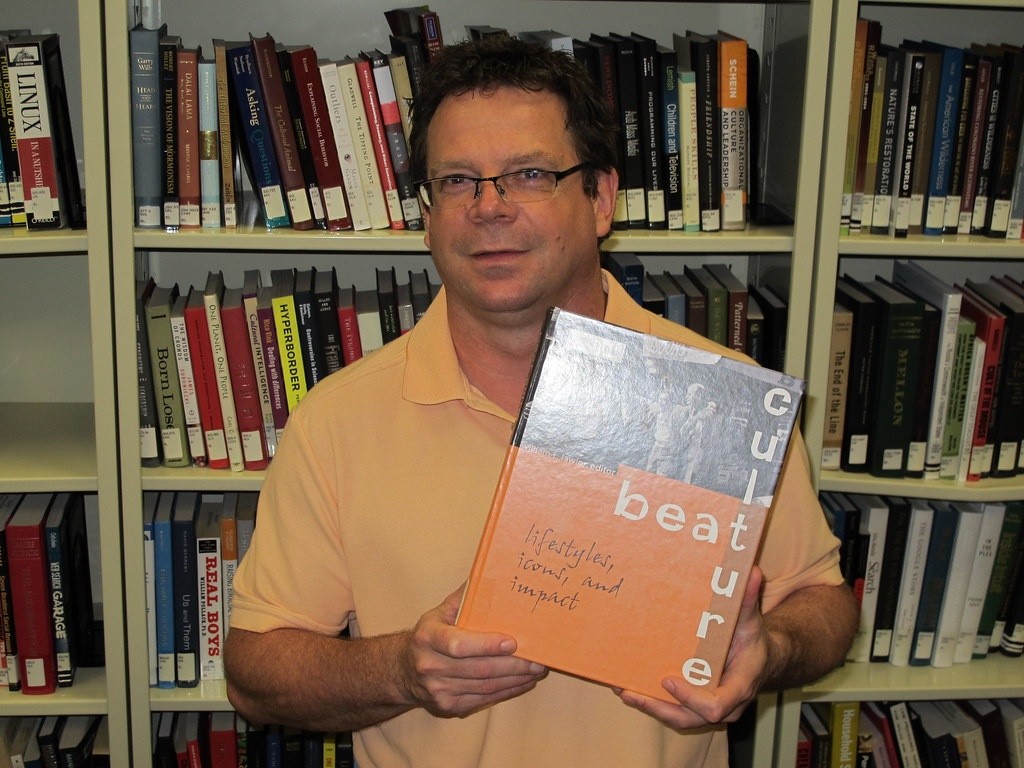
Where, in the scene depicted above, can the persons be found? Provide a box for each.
[216,28,860,768]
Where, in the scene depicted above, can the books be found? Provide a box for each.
[786,17,1024,768]
[0,19,789,768]
[449,302,808,712]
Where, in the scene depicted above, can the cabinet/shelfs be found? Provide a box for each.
[0,0,1020,768]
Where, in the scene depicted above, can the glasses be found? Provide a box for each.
[412,159,591,209]
